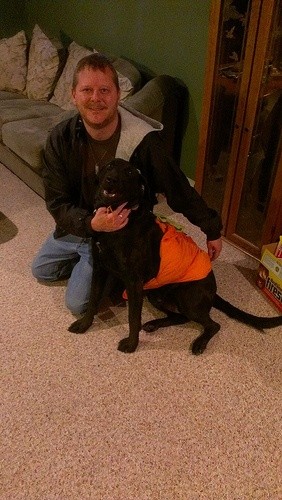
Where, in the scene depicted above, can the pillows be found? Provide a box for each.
[23,24,60,101]
[93,49,134,102]
[50,41,95,114]
[0,30,28,93]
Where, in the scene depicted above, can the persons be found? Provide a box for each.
[31,53,224,314]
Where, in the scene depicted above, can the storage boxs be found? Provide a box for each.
[256,242,282,313]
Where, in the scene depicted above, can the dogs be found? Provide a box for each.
[66,157,282,356]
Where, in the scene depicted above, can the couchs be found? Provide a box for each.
[0,27,173,200]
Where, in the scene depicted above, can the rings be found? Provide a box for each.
[119,214,123,219]
